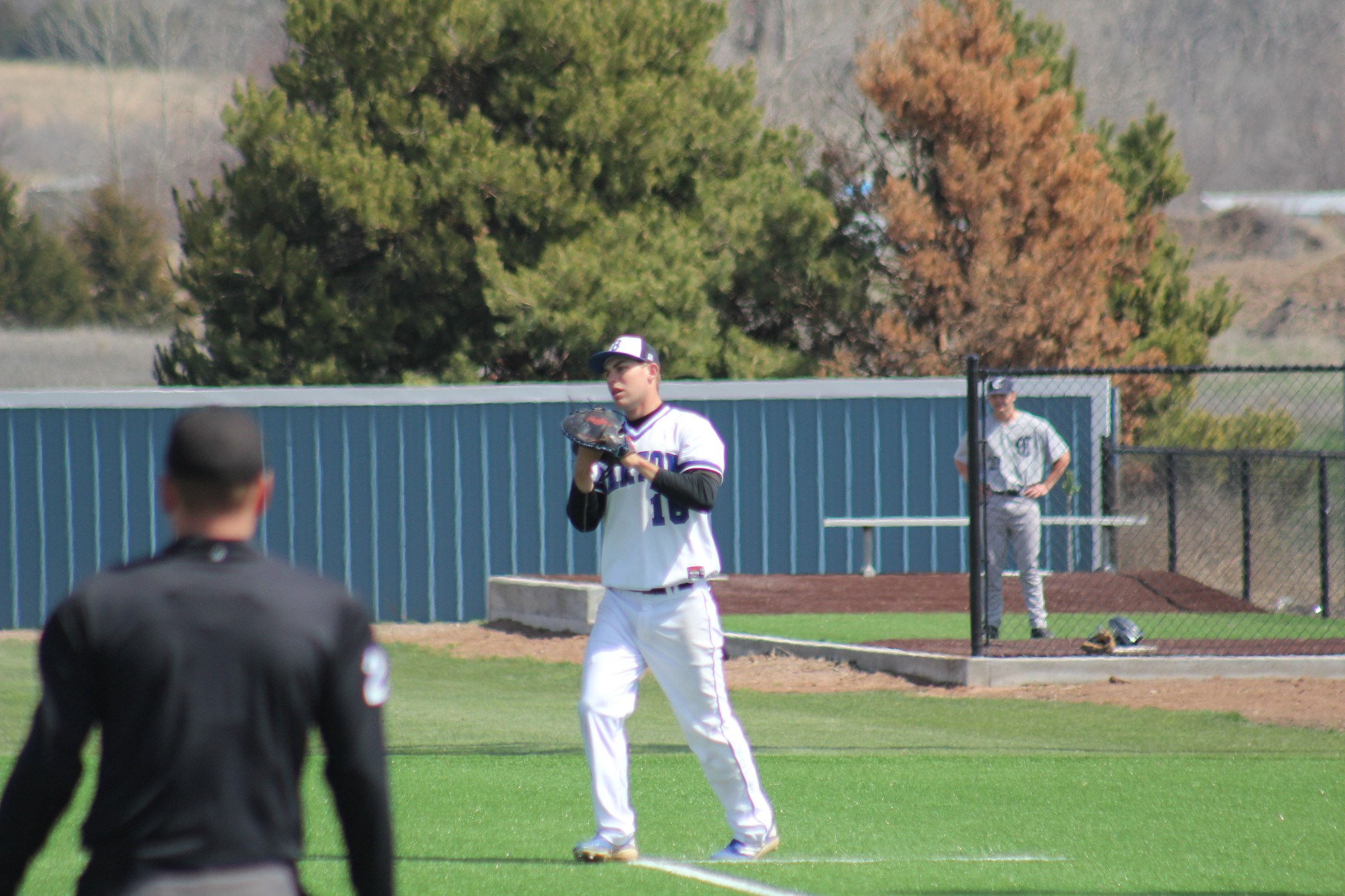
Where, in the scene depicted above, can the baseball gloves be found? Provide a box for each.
[1107,616,1145,646]
[1082,629,1114,655]
[560,396,637,459]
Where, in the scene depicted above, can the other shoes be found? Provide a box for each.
[1031,628,1054,640]
[981,625,998,640]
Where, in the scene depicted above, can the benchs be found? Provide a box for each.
[820,517,1148,577]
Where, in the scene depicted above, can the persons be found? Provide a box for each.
[565,337,779,865]
[952,376,1070,643]
[0,406,396,896]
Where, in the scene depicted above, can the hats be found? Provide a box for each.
[588,334,660,374]
[986,377,1015,396]
[165,404,263,482]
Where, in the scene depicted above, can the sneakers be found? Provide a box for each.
[572,833,638,862]
[708,827,780,862]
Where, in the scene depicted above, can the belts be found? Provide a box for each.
[993,489,1020,497]
[627,581,694,594]
